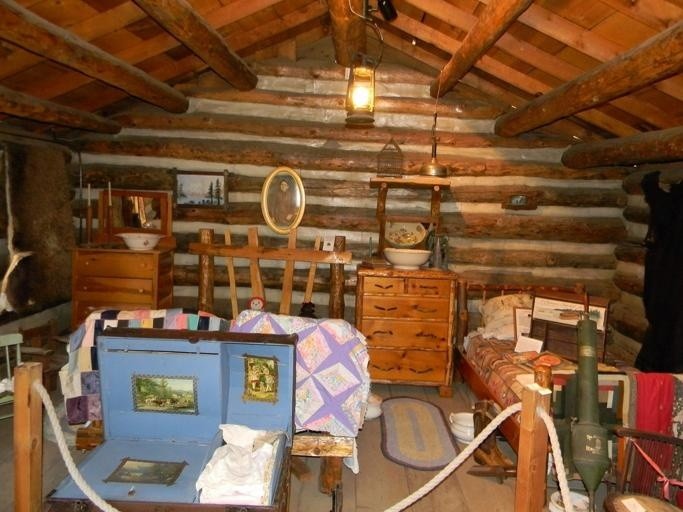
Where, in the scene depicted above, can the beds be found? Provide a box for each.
[454,277,683,512]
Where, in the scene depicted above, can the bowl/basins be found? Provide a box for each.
[383,247,433,271]
[448,412,474,445]
[365,393,383,419]
[113,232,165,251]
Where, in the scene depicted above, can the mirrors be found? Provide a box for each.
[100,189,173,240]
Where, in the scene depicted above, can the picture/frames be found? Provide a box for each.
[260,166,306,235]
[172,167,229,210]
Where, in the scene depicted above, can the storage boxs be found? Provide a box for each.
[42,327,298,511]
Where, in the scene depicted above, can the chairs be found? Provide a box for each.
[603,425,683,512]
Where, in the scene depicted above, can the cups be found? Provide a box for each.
[510,195,527,205]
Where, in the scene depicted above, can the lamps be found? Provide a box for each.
[343,11,385,130]
[419,73,447,179]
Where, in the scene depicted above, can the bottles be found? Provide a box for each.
[433,236,442,267]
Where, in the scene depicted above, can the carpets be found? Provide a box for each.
[380,393,462,473]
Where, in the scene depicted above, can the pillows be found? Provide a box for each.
[478,290,539,341]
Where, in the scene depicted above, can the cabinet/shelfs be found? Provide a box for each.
[354,177,462,398]
[70,250,174,335]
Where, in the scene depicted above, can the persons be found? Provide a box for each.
[269,178,297,226]
[248,363,260,391]
[261,362,275,392]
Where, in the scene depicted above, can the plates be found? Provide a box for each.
[384,221,426,247]
[550,490,596,512]
